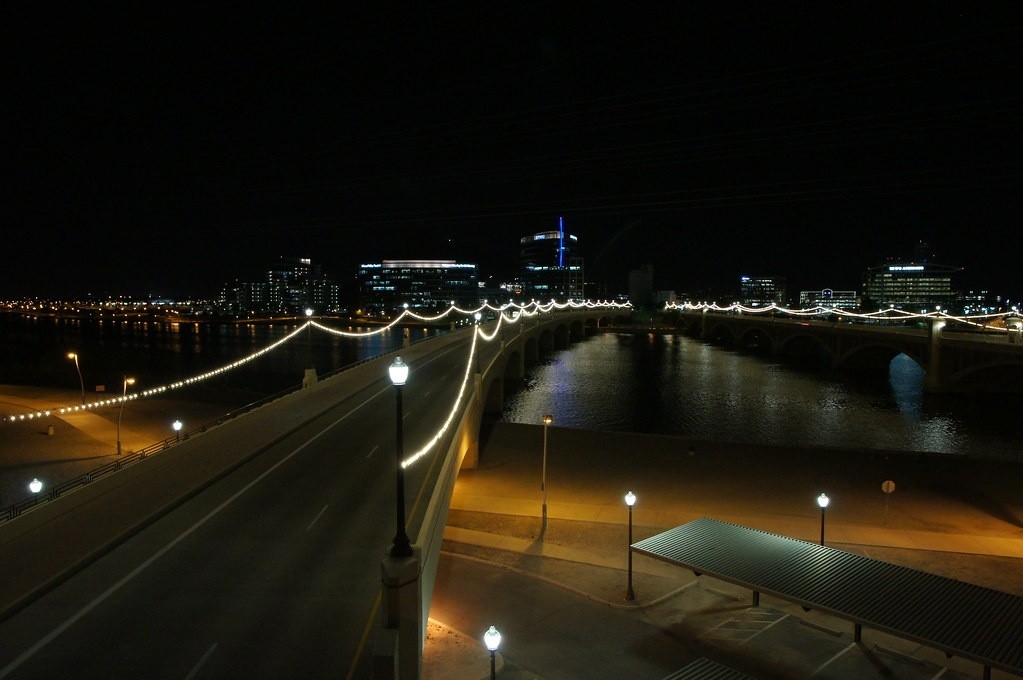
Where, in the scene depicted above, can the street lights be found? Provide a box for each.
[816,491,831,545]
[624,491,637,602]
[299,307,320,388]
[385,352,415,558]
[29,477,43,503]
[115,375,135,454]
[173,420,184,442]
[482,624,502,680]
[539,412,553,496]
[66,351,86,404]
